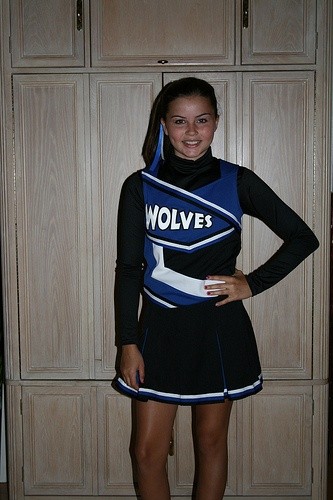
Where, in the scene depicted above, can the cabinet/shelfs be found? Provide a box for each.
[1,2,331,497]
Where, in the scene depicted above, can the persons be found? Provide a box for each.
[111,77,321,500]
